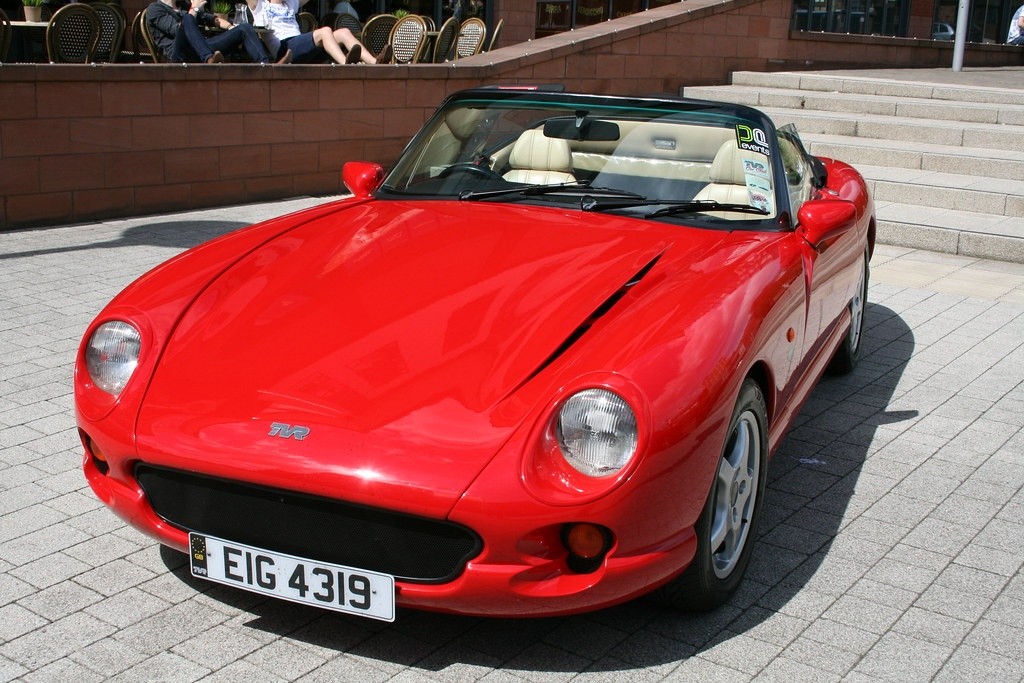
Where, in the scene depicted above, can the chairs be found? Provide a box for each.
[419,15,436,63]
[334,13,362,34]
[360,13,400,58]
[388,14,428,64]
[692,139,773,221]
[502,128,579,186]
[453,18,487,60]
[0,2,159,64]
[320,12,341,30]
[299,12,318,32]
[488,19,504,52]
[433,16,461,64]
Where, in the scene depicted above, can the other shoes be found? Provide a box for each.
[376,45,392,64]
[207,50,224,63]
[276,48,292,64]
[345,44,361,64]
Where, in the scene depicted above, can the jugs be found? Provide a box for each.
[233,4,248,26]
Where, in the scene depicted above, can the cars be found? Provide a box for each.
[931,22,956,41]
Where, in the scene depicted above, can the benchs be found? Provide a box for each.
[570,119,812,209]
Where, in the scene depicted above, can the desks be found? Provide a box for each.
[356,31,464,63]
[201,29,275,63]
[2,21,56,63]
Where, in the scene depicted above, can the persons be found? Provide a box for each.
[146,0,293,64]
[1007,5,1024,45]
[246,0,393,64]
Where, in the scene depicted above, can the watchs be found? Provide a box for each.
[190,7,200,12]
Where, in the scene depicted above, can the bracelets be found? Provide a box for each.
[227,25,232,29]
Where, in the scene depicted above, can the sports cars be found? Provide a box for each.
[74,83,878,624]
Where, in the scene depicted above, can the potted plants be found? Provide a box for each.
[212,1,234,29]
[22,0,45,22]
